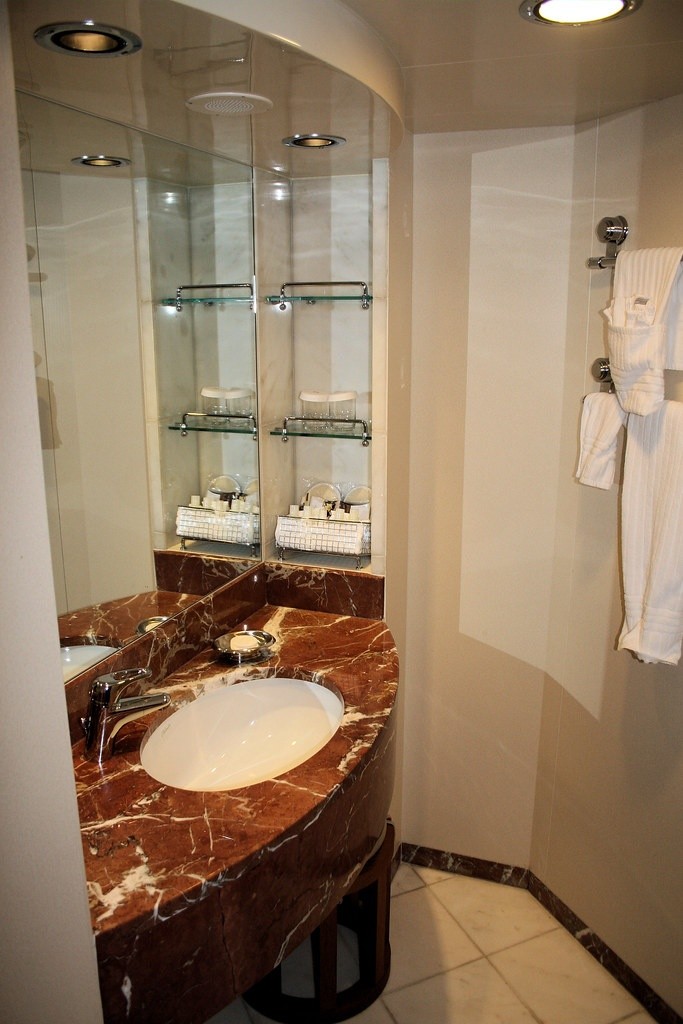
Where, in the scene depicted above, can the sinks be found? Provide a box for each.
[59,634,122,688]
[135,655,349,795]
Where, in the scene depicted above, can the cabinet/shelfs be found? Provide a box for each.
[265,281,372,447]
[158,282,257,441]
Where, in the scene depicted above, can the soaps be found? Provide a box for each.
[229,634,261,654]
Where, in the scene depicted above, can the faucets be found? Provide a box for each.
[83,666,171,762]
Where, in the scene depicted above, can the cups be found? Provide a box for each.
[302,399,329,432]
[227,395,252,428]
[203,398,226,425]
[329,398,356,432]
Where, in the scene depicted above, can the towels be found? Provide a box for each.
[575,388,627,492]
[603,244,683,668]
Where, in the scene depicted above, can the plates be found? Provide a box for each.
[343,486,372,504]
[209,476,241,500]
[242,479,258,496]
[301,483,341,518]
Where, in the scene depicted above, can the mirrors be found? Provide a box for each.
[15,86,263,679]
